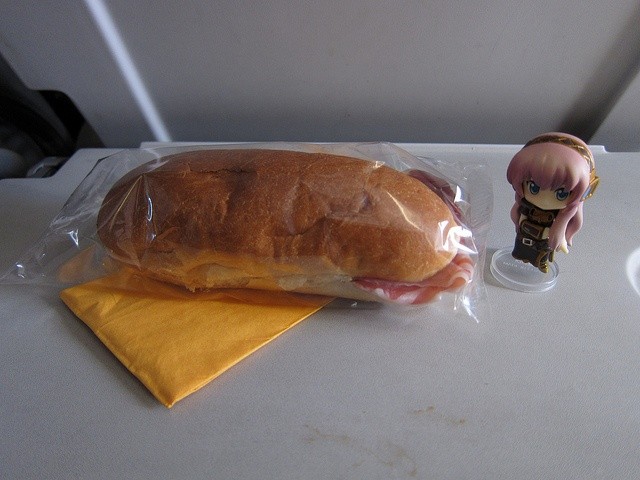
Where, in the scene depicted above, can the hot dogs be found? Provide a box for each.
[96,149,477,310]
